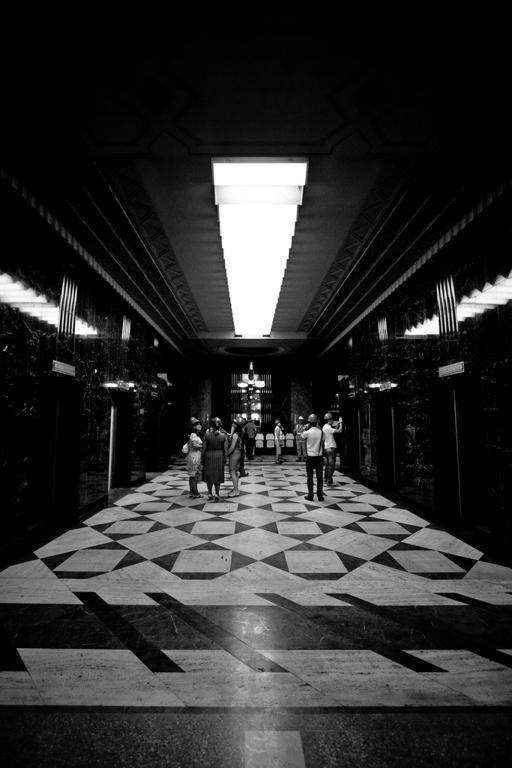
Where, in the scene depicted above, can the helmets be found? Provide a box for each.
[275,420,280,424]
[299,413,332,423]
[190,413,246,427]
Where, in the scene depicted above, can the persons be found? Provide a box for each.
[274,419,288,465]
[292,412,344,503]
[183,411,257,501]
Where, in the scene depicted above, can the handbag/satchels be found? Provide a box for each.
[181,443,190,453]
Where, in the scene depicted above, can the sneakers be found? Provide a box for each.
[318,496,324,501]
[305,495,313,501]
[190,473,248,502]
